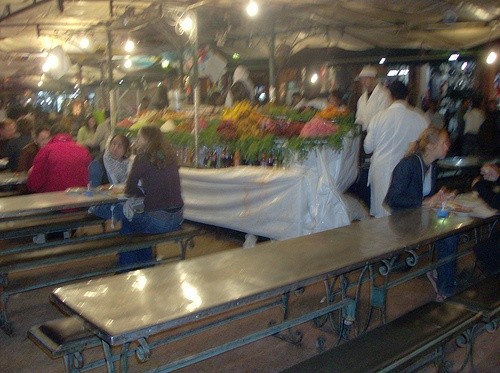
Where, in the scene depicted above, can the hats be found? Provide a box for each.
[358,63,378,77]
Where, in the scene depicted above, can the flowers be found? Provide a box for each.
[113,99,361,168]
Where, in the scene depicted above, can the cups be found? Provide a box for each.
[32,233,46,245]
[63,231,71,240]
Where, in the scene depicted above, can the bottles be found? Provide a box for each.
[176,145,285,168]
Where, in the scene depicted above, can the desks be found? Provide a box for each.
[50,184,500,373]
[0,171,128,253]
[103,124,361,241]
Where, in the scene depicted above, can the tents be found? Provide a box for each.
[0,0,500,86]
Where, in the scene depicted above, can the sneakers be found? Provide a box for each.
[33,236,46,244]
[426,269,447,298]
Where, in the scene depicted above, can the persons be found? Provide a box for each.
[87,129,134,223]
[0,61,500,303]
[23,123,94,244]
[118,127,185,273]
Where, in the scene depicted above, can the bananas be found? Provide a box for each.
[219,99,259,122]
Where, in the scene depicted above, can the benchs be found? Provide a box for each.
[27,282,499,373]
[1,223,200,336]
